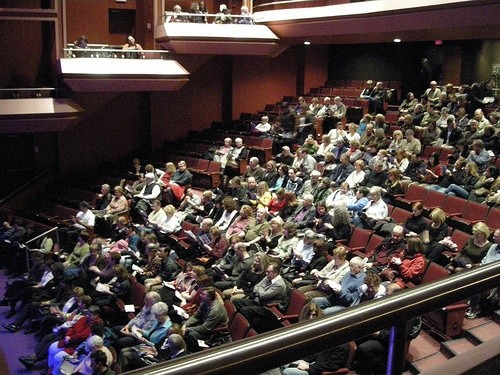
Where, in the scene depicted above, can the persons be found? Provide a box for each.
[214,4,233,24]
[234,6,254,24]
[122,36,146,59]
[0,0,500,375]
[72,36,93,58]
[190,1,209,23]
[168,5,187,23]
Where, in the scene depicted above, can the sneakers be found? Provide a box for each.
[0,298,9,306]
[23,328,35,335]
[1,323,16,333]
[19,355,37,371]
[7,274,16,279]
[5,308,16,319]
[464,307,480,320]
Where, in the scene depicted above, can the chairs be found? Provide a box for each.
[0,78,500,375]
